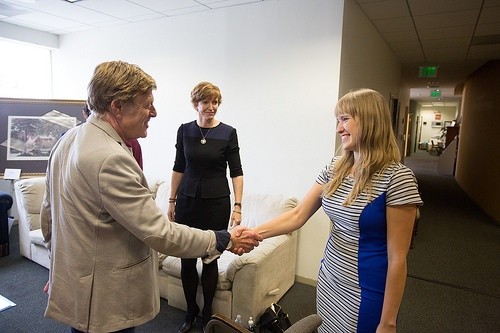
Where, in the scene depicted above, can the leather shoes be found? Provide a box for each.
[177,314,196,333]
[197,315,212,333]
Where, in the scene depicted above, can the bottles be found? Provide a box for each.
[247,316,254,333]
[235,314,243,326]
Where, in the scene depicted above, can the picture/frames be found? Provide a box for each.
[0,98,87,179]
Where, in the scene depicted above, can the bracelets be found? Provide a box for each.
[169,198,175,202]
[233,202,242,213]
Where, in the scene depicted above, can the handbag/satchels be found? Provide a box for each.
[248,303,291,333]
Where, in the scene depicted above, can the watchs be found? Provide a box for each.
[226,239,233,250]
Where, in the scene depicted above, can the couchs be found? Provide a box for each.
[14,176,299,328]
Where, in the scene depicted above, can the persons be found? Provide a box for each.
[233,88,423,333]
[83,104,143,170]
[40,61,263,332]
[168,81,243,333]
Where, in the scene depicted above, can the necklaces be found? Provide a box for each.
[196,118,214,145]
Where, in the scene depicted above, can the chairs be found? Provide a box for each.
[205,313,322,333]
